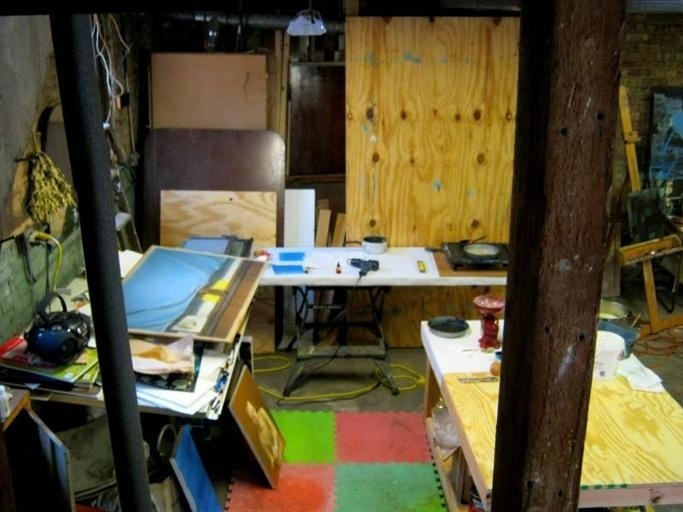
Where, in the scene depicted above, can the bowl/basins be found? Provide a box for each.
[596,319,641,359]
[362,237,389,254]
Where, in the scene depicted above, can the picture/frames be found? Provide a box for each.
[646,86,682,181]
[228,364,286,490]
[121,245,265,344]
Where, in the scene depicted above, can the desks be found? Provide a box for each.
[420,320,683,512]
[0,249,251,418]
[251,246,513,395]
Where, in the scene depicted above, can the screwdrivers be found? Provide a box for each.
[336,261,341,273]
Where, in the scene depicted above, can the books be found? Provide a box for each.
[0,336,103,402]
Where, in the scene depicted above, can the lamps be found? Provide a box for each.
[286,0,326,37]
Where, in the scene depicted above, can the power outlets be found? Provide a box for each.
[17,227,36,254]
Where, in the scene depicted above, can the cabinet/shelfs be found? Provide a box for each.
[0,389,73,512]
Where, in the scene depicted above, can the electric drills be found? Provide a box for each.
[346,258,378,276]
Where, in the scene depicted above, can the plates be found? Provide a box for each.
[428,315,471,339]
